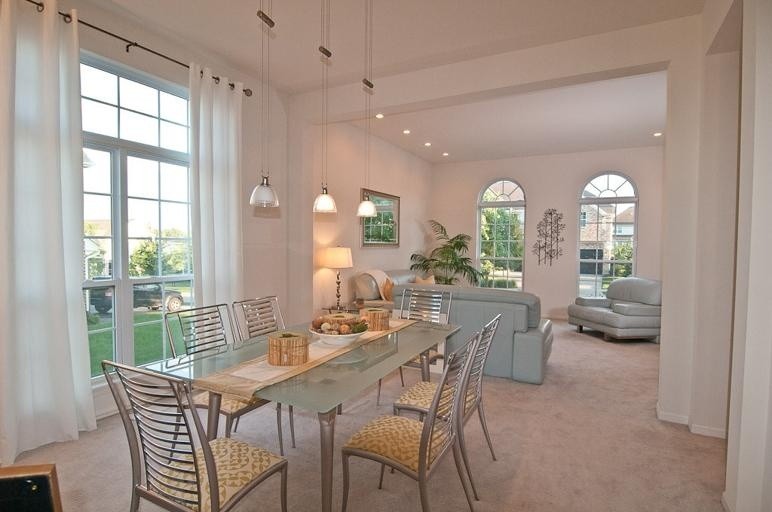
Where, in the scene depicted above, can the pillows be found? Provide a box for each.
[384,278,393,301]
[415,275,436,285]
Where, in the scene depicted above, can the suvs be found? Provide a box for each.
[89,275,183,313]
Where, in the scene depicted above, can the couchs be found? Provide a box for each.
[353,269,430,311]
[381,282,554,386]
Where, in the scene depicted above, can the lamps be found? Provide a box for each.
[356,1,378,218]
[325,244,354,310]
[249,1,281,209]
[313,1,339,215]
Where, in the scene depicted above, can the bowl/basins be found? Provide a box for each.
[308,328,367,347]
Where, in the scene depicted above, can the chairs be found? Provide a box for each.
[566,275,662,342]
